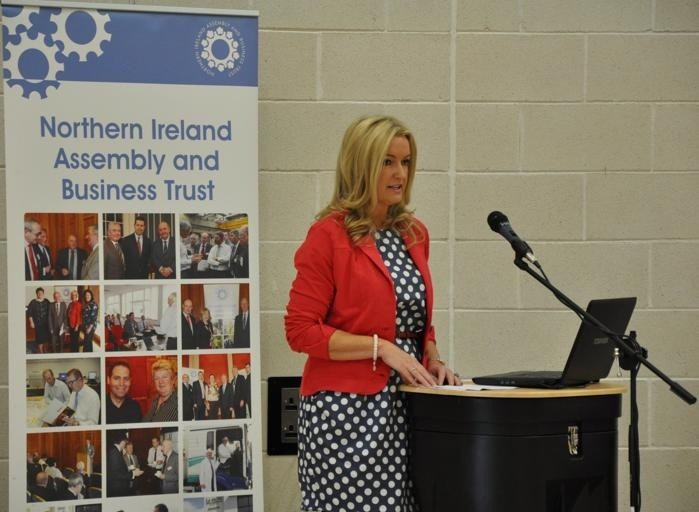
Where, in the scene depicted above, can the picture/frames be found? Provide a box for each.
[267,376,303,455]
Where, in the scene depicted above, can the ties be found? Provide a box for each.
[164,240,167,253]
[43,247,49,266]
[201,244,205,257]
[154,449,157,461]
[28,243,39,280]
[115,243,123,264]
[70,250,74,272]
[187,315,191,329]
[138,237,141,256]
[243,314,245,329]
[130,456,133,465]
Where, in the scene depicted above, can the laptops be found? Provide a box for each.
[472,295,639,389]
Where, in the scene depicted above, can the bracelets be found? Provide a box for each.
[373,334,378,371]
[429,356,447,365]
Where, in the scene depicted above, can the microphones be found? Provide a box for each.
[487,211,541,269]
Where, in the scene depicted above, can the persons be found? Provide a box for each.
[104,293,177,352]
[102,218,176,279]
[42,368,100,427]
[141,359,178,422]
[105,361,142,424]
[182,363,251,422]
[27,439,96,502]
[24,218,99,280]
[180,221,249,278]
[284,115,462,512]
[28,287,99,354]
[106,429,179,497]
[181,298,250,349]
[182,430,251,494]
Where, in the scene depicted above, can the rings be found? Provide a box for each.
[454,372,460,377]
[409,368,415,372]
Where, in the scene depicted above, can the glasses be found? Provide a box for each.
[66,380,78,386]
